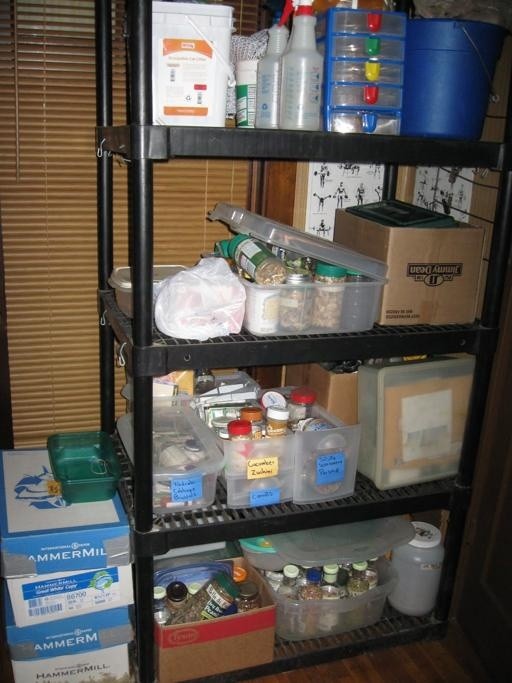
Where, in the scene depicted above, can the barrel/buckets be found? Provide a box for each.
[403,18,497,135]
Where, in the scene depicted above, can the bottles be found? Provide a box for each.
[227,234,371,338]
[275,556,380,636]
[211,385,343,504]
[386,520,446,622]
[152,572,260,644]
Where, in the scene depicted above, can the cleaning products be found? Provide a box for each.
[257,21,287,128]
[277,0,323,131]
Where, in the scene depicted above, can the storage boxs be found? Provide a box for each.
[0,435,137,682]
[153,513,416,683]
[117,352,478,514]
[108,203,485,343]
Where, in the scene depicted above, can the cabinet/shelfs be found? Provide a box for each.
[86,0,512,683]
